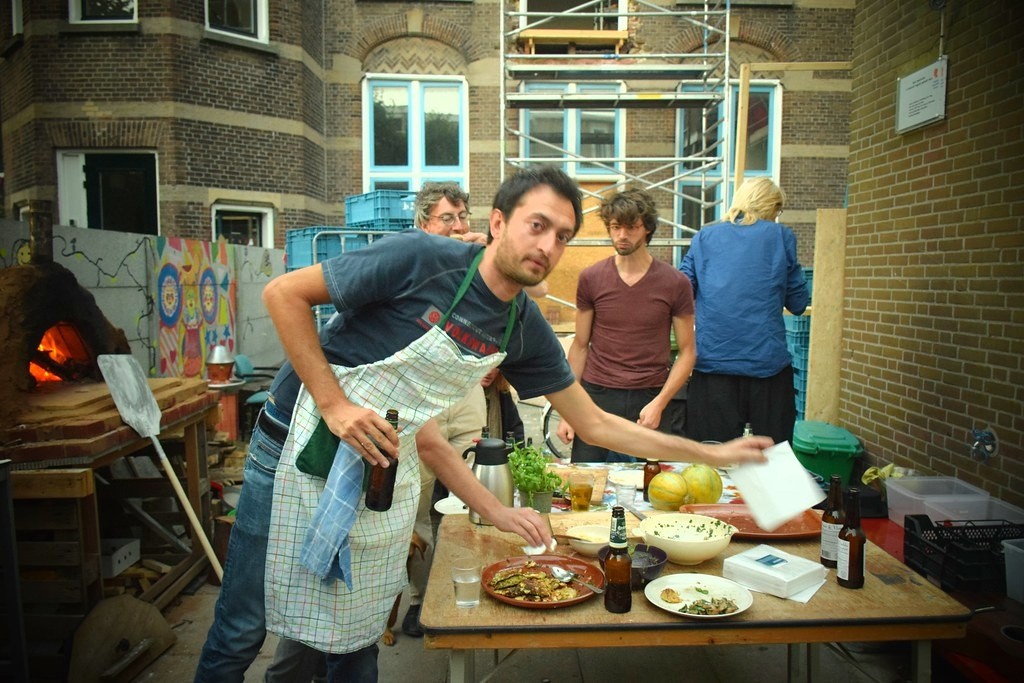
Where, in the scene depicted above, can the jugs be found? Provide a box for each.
[462,438,515,525]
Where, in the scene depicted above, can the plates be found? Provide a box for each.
[480,555,605,608]
[607,470,646,489]
[644,573,753,617]
[716,466,734,471]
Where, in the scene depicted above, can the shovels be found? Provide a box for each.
[97,354,224,586]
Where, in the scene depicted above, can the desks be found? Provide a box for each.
[0,382,221,628]
[417,455,972,683]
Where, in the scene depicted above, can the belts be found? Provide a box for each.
[258,410,289,446]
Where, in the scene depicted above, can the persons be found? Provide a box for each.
[193,169,775,683]
[677,177,809,449]
[380,182,553,646]
[556,188,697,464]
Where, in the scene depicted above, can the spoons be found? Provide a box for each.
[551,565,604,593]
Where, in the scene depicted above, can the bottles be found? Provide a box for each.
[604,507,632,613]
[643,457,661,503]
[820,473,848,567]
[837,488,866,589]
[364,409,398,512]
[742,421,754,436]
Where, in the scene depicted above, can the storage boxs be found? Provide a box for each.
[66,537,141,578]
[283,188,421,340]
[999,540,1024,602]
[881,470,989,525]
[898,511,1024,606]
[782,312,811,419]
[802,267,812,300]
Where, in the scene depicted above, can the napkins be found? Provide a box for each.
[726,440,825,533]
[725,542,827,606]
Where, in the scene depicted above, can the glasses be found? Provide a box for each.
[777,207,784,216]
[429,210,473,225]
[606,223,644,232]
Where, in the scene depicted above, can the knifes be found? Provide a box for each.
[620,501,647,521]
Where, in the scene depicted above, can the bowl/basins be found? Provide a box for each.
[640,514,740,565]
[565,525,610,558]
[597,543,668,590]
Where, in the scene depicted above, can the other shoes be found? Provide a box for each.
[401,604,424,637]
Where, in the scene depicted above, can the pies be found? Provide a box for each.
[488,567,576,604]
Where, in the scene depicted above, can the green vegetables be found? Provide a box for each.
[653,519,732,538]
[679,587,726,615]
[507,438,569,506]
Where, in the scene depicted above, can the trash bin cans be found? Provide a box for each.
[792,420,864,486]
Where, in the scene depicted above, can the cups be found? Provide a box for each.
[451,558,482,608]
[568,473,593,512]
[616,483,636,512]
[701,440,722,445]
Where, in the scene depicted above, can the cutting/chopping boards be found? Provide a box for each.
[546,468,609,505]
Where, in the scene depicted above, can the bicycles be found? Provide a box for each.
[539,401,573,459]
